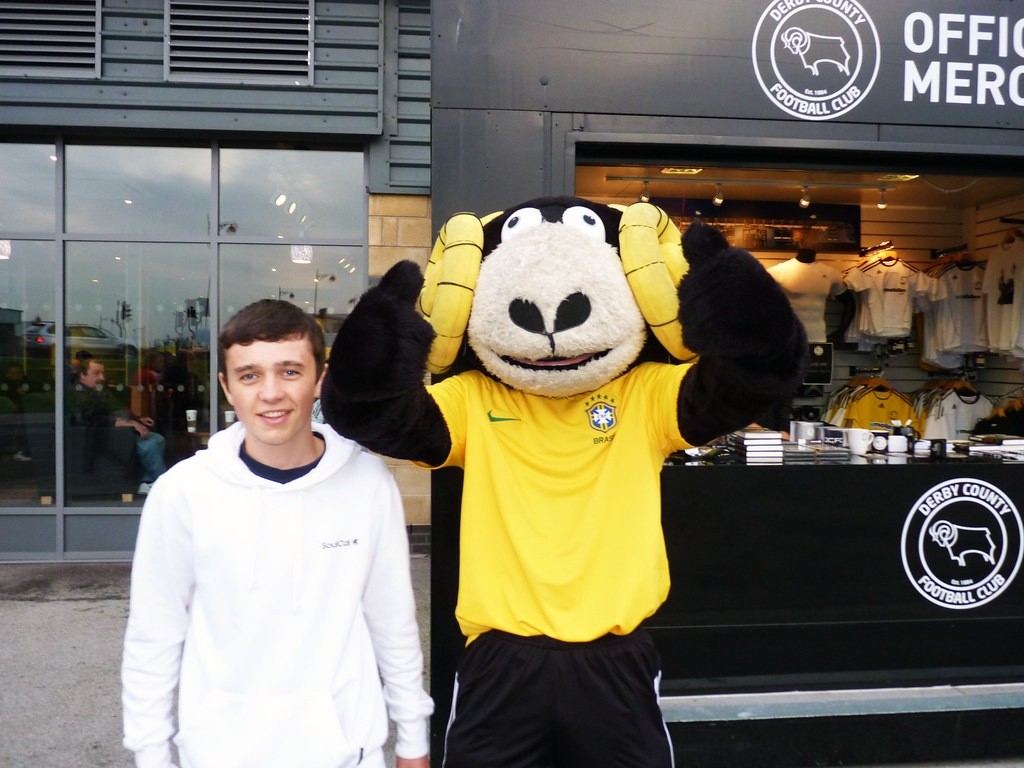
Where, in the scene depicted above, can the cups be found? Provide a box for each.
[849,429,874,455]
[186,409,197,433]
[870,430,890,453]
[224,411,236,428]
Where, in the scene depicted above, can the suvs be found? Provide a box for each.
[21,321,138,363]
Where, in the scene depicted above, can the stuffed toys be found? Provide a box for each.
[321,202,809,768]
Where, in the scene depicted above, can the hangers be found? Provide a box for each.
[878,241,897,259]
[935,372,977,395]
[846,366,893,392]
[988,389,1024,420]
[1002,219,1024,245]
[944,243,973,266]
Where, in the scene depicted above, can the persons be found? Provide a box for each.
[121,299,437,768]
[66,360,167,495]
[0,344,94,462]
[128,350,167,424]
[765,249,844,344]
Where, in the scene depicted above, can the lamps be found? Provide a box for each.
[878,188,887,209]
[712,184,724,206]
[799,186,811,209]
[641,182,649,202]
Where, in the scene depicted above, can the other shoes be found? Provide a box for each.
[138,483,151,494]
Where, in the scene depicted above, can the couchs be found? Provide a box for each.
[25,393,141,504]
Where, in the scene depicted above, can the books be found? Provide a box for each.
[726,425,849,465]
